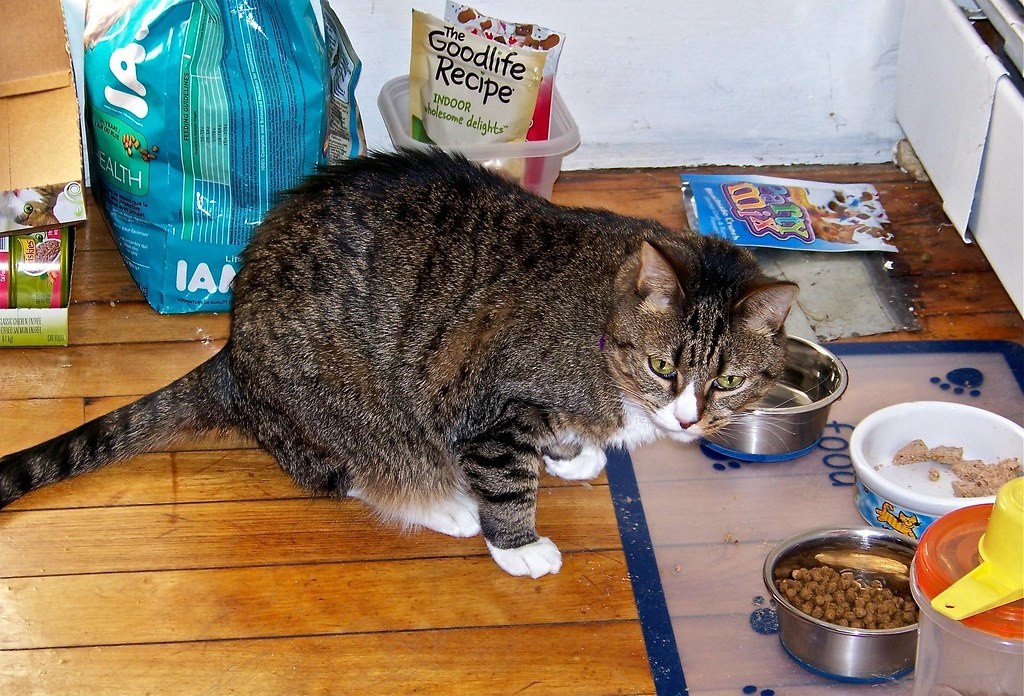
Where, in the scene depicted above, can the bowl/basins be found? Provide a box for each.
[698,336,848,462]
[850,402,1023,538]
[765,527,921,683]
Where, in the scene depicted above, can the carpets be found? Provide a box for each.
[606,336,1024,696]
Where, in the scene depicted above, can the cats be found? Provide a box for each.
[0,146,796,579]
[0,180,81,231]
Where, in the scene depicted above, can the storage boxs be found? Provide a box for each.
[380,72,580,201]
[0,0,91,348]
[887,0,1024,320]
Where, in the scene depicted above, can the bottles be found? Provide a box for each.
[909,503,1024,696]
